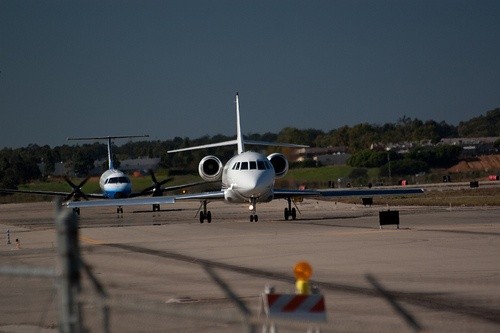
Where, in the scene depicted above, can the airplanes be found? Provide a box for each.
[63,92,427,224]
[2,132,223,215]
